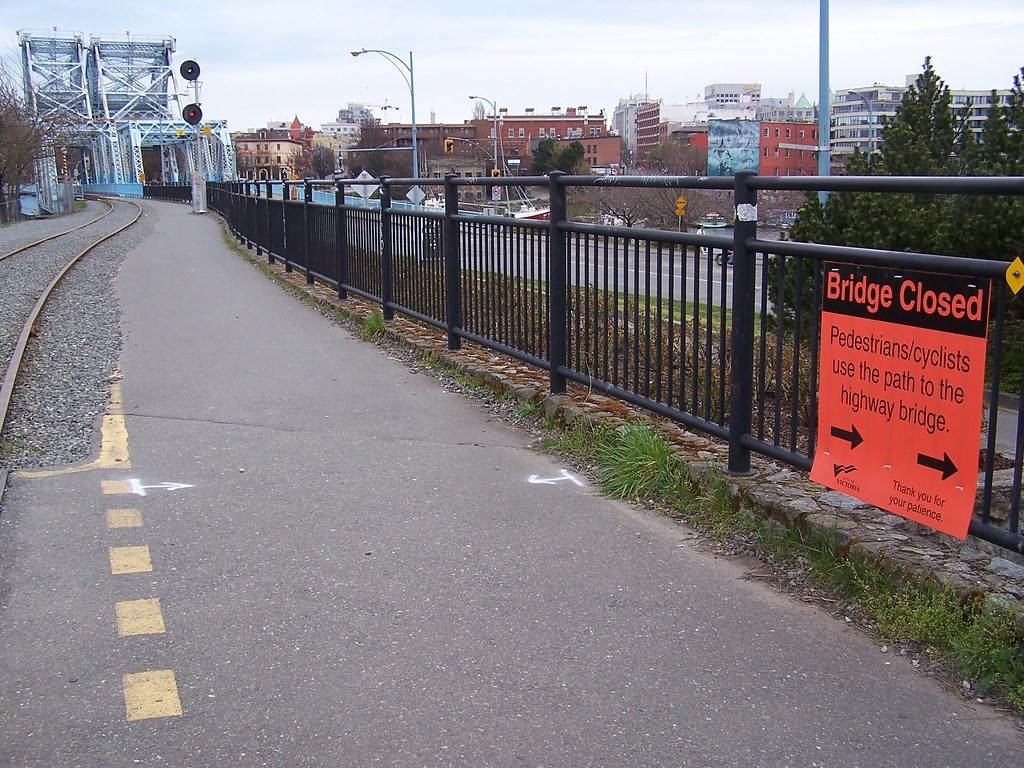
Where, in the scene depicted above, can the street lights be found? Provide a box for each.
[468,95,498,167]
[351,48,419,177]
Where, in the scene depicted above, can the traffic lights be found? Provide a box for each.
[492,170,501,178]
[181,61,201,81]
[182,104,203,125]
[445,139,454,154]
